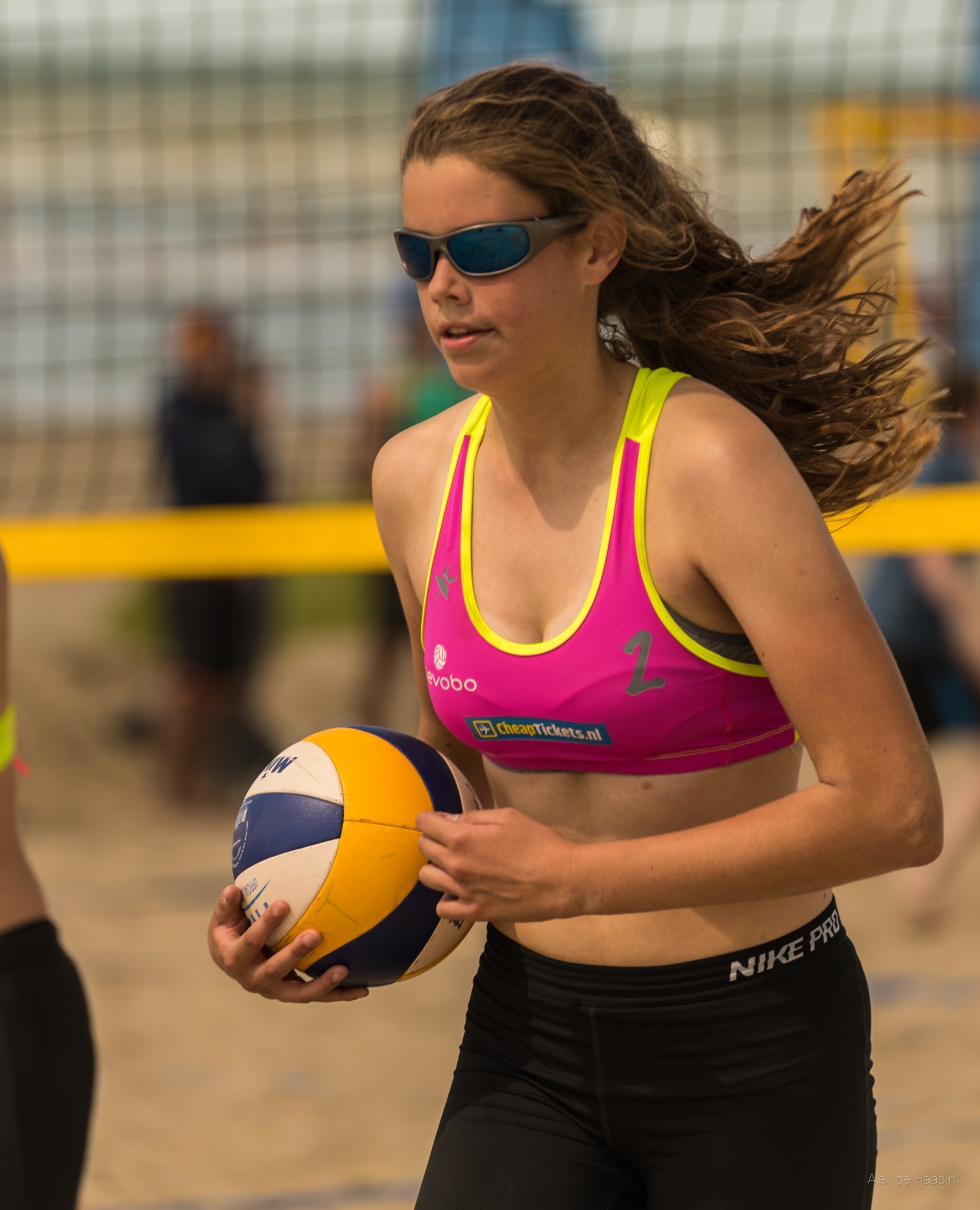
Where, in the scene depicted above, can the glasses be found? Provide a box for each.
[391,215,595,284]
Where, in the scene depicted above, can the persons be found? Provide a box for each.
[154,280,484,822]
[0,559,95,1210]
[845,355,980,924]
[206,62,946,1209]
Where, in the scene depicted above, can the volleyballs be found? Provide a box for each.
[230,726,485,985]
